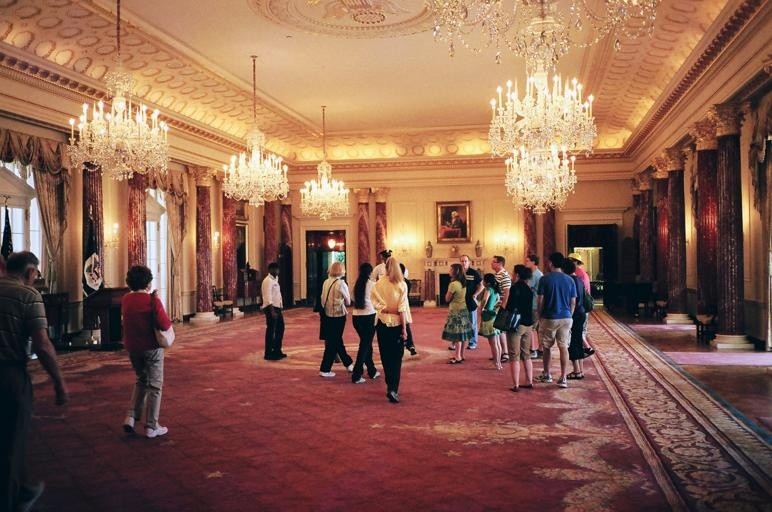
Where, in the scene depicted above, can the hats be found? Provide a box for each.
[567,253,583,264]
[380,250,392,257]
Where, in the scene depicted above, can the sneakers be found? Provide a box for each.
[347,363,380,383]
[530,349,543,358]
[582,347,594,358]
[122,416,134,432]
[145,424,168,438]
[533,374,553,383]
[20,480,45,510]
[320,370,335,378]
[556,376,567,388]
[387,391,398,402]
[265,354,287,360]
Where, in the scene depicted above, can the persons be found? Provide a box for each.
[118,264,176,442]
[370,256,411,402]
[437,250,597,395]
[349,263,386,385]
[320,262,356,377]
[259,261,289,360]
[312,270,351,363]
[2,249,67,510]
[394,262,419,361]
[368,247,392,278]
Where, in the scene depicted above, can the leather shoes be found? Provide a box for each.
[510,386,519,390]
[519,384,533,388]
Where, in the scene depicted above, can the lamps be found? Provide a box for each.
[68,0,171,181]
[219,56,289,208]
[299,105,350,220]
[428,1,660,216]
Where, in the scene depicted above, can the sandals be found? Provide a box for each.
[566,371,584,379]
[488,350,509,370]
[448,356,464,364]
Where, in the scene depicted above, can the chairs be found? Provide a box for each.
[406,279,421,306]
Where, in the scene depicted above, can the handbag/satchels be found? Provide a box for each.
[492,308,522,333]
[154,322,176,348]
[584,290,595,313]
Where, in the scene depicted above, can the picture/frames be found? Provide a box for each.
[435,201,473,244]
